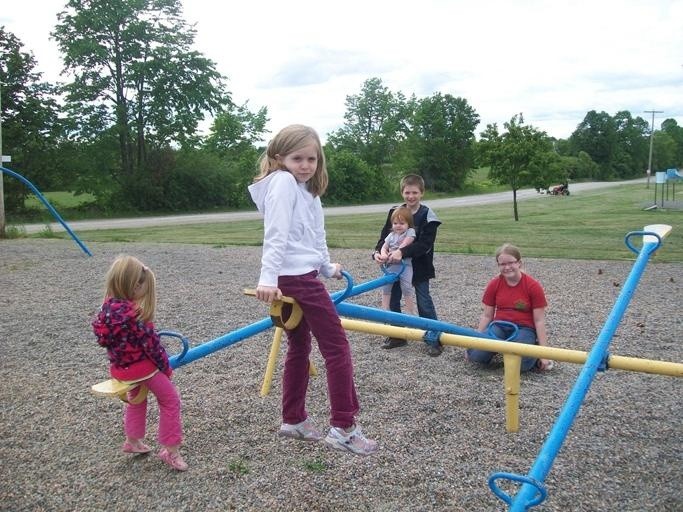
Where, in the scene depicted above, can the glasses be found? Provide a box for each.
[499,261,516,265]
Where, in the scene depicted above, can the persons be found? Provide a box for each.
[92,255,188,472]
[248,124,380,457]
[371,174,442,356]
[466,244,554,373]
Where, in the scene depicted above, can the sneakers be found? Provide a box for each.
[279,417,323,441]
[325,423,378,455]
[158,448,188,471]
[545,359,553,371]
[123,442,152,453]
[429,347,443,357]
[380,337,407,349]
